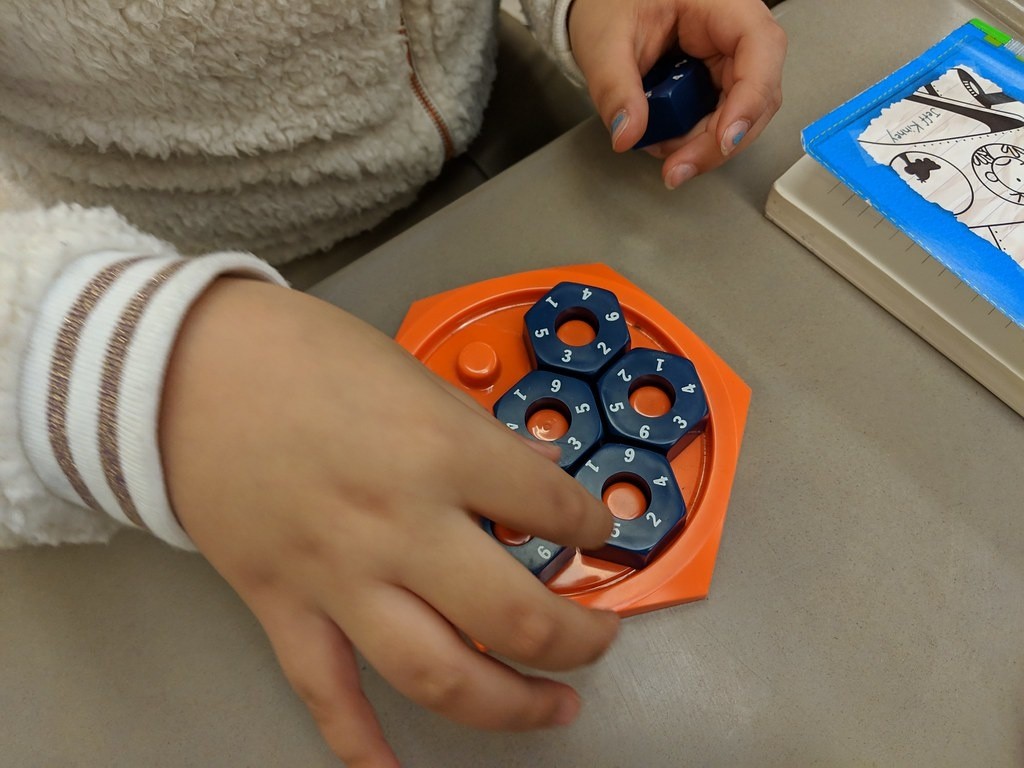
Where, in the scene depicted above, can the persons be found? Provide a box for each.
[1,1,792,766]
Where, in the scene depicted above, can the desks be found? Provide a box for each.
[0,0,1024,768]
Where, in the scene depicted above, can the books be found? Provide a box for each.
[762,12,1022,427]
